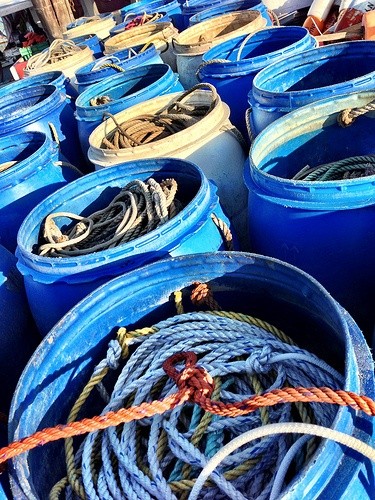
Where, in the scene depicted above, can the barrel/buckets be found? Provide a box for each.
[1,0,374,500]
